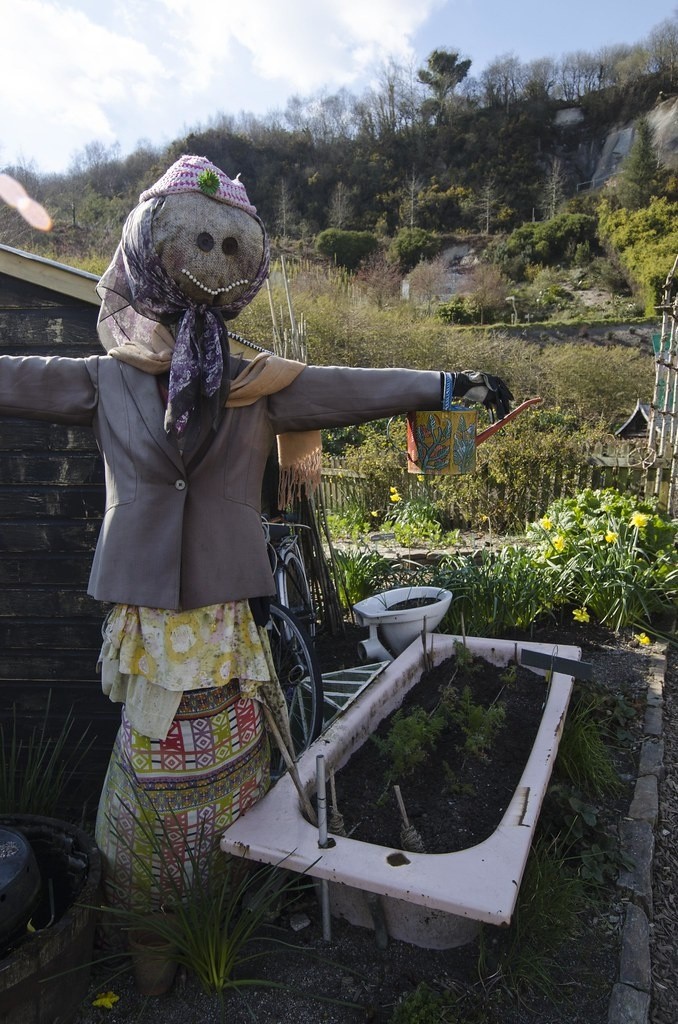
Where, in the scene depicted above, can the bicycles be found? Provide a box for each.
[260,515,323,785]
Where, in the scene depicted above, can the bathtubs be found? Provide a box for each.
[217,631,582,950]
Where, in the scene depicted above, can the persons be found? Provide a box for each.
[1,154,515,920]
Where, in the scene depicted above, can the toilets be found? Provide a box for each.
[352,586,453,665]
